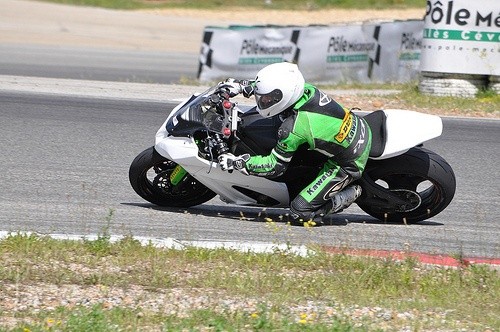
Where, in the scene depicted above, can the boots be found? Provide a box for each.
[327,184,361,215]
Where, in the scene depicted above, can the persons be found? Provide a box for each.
[218,62,372,224]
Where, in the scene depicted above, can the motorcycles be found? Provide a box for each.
[129,82,457,224]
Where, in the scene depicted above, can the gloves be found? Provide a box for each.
[216,78,254,99]
[216,152,251,177]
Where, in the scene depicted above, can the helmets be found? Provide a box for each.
[251,60,305,117]
